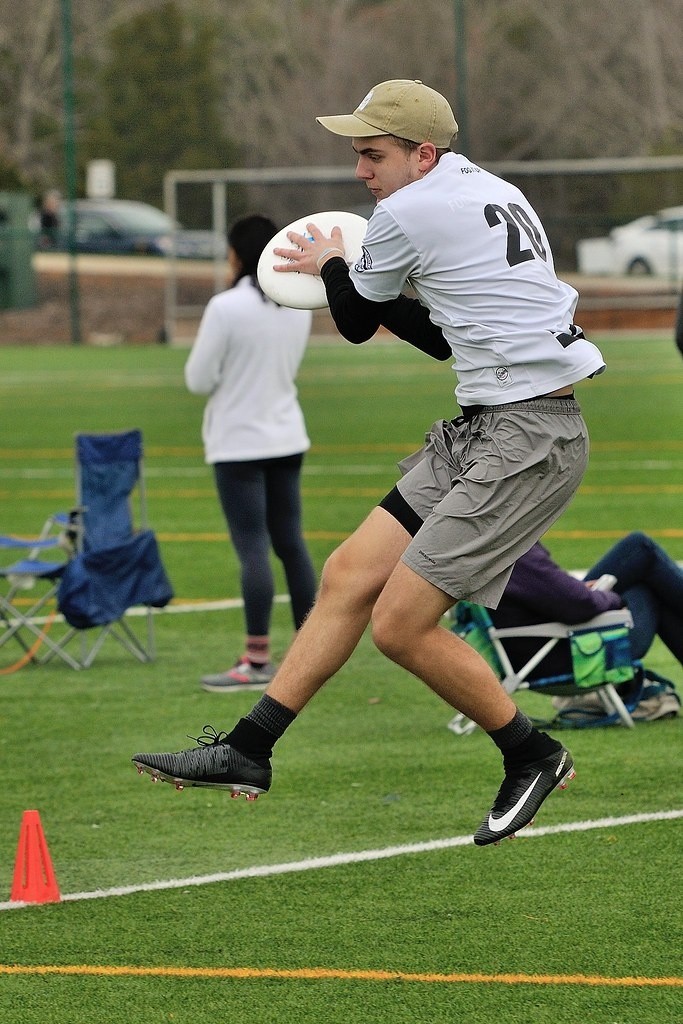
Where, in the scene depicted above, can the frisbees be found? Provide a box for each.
[257,213,372,307]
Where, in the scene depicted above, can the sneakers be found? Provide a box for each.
[131,724,272,802]
[473,731,577,847]
[199,656,280,693]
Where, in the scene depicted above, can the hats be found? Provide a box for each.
[315,79,459,148]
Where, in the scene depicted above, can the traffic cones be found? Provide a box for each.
[9,809,60,904]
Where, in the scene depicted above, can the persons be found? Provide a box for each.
[184,216,312,691]
[40,189,62,248]
[133,78,605,848]
[485,532,683,678]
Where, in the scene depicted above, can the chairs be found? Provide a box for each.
[447,594,635,736]
[0,428,175,669]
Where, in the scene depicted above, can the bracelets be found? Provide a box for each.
[316,246,343,269]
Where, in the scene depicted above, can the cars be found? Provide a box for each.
[577,202,683,282]
[39,199,231,262]
